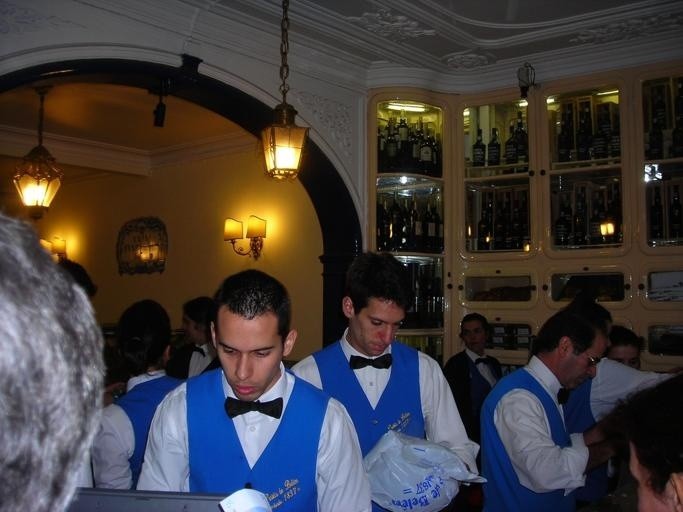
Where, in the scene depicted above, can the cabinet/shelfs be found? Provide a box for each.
[633,62,682,256]
[367,87,451,365]
[453,263,539,377]
[636,254,682,370]
[537,70,636,259]
[542,259,635,339]
[455,89,539,261]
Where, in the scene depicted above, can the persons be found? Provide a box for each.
[169,297,222,380]
[443,311,503,511]
[89,297,188,490]
[56,257,99,301]
[137,267,372,512]
[289,248,480,512]
[0,210,110,512]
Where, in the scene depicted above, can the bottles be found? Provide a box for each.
[372,108,442,364]
[467,86,683,249]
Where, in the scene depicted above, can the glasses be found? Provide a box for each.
[585,354,602,368]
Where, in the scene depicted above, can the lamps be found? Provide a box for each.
[247,0,312,182]
[222,207,269,261]
[11,84,68,226]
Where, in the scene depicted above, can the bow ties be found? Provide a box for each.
[475,357,491,365]
[348,353,394,369]
[558,387,570,405]
[224,397,283,422]
